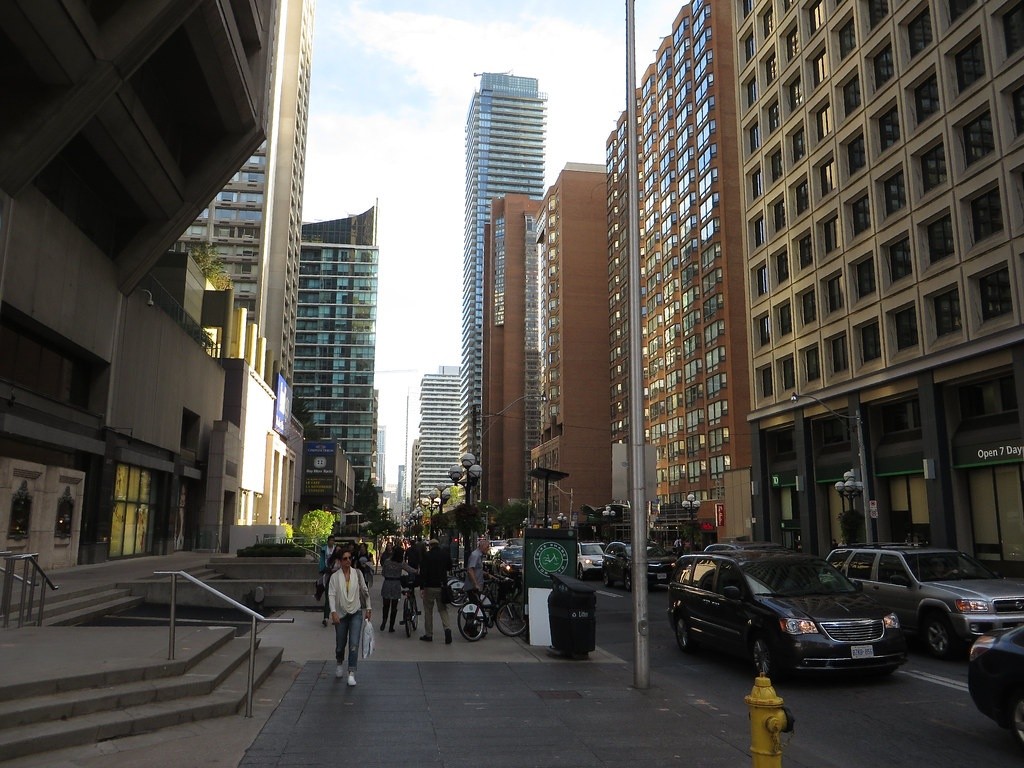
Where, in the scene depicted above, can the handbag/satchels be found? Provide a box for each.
[441,585,452,604]
[322,560,335,588]
[362,618,375,659]
[355,570,368,602]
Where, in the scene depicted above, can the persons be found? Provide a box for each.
[379,537,452,644]
[328,548,372,686]
[320,535,374,626]
[464,540,490,637]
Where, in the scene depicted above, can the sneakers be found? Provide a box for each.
[336,665,343,678]
[348,675,356,686]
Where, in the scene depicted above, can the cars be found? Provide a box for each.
[966,622,1024,740]
[702,540,795,560]
[667,550,908,681]
[478,537,524,570]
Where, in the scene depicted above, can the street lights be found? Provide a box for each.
[420,486,441,540]
[836,471,865,510]
[448,453,482,573]
[409,507,424,542]
[436,482,452,540]
[602,505,616,541]
[548,482,573,530]
[557,512,568,529]
[681,494,701,551]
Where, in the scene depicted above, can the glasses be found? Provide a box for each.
[342,556,351,561]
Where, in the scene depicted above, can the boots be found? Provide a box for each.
[389,608,398,632]
[380,607,388,631]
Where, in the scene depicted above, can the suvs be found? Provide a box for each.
[819,543,1024,658]
[601,540,677,591]
[576,541,613,582]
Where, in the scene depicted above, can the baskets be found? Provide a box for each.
[401,575,416,587]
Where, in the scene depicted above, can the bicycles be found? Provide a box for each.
[447,563,472,608]
[399,572,423,638]
[456,577,528,642]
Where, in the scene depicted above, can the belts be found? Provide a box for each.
[385,577,399,581]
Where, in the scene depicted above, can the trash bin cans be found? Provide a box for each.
[547,572,596,652]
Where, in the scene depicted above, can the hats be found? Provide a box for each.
[429,539,439,545]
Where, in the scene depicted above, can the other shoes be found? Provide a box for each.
[472,631,485,638]
[445,629,452,644]
[419,634,432,641]
[463,629,475,637]
[322,619,328,626]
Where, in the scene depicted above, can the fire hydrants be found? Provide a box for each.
[743,671,794,768]
